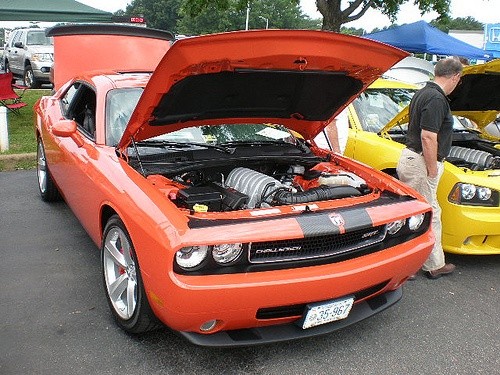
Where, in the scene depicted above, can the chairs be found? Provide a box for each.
[0,72,28,115]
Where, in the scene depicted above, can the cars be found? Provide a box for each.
[3,27,57,87]
[34,20,436,346]
[342,57,500,255]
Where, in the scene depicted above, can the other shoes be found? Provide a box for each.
[407,274,416,281]
[428,264,456,279]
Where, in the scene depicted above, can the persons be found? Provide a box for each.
[396,57,464,280]
[459,56,469,66]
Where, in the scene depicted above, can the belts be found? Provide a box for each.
[406,146,446,162]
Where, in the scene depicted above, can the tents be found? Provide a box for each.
[361,20,494,60]
[0,0,115,23]
